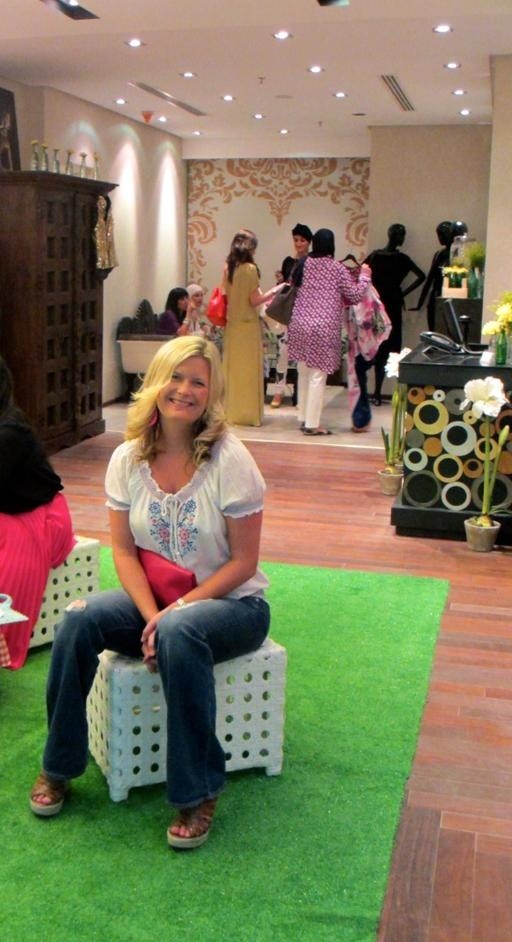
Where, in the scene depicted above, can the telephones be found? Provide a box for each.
[419,330,461,352]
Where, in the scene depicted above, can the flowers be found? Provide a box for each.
[458,378,512,526]
[378,345,412,494]
[30,140,101,181]
[441,265,468,277]
[480,290,512,365]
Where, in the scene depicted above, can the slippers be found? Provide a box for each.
[303,428,332,436]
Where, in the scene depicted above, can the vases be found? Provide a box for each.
[447,274,465,289]
[467,267,478,299]
[463,514,501,552]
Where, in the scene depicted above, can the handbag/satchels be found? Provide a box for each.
[265,259,299,325]
[205,287,228,327]
[136,547,198,610]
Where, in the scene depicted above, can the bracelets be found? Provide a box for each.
[177,597,185,606]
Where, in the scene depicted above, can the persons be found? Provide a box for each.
[217,229,292,426]
[355,224,428,406]
[184,283,223,355]
[286,229,372,436]
[406,218,470,337]
[1,356,75,672]
[156,287,196,336]
[270,223,314,408]
[30,336,271,849]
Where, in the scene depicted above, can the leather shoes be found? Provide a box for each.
[271,398,281,408]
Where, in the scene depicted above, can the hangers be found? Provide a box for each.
[338,240,364,269]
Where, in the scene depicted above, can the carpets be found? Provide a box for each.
[96,376,395,452]
[1,544,455,939]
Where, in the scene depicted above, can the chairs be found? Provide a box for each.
[113,296,176,375]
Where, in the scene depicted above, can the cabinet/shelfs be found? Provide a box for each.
[0,169,121,459]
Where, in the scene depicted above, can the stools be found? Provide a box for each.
[21,530,104,652]
[90,632,289,803]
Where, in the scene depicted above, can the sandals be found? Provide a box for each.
[27,770,66,816]
[167,795,216,849]
